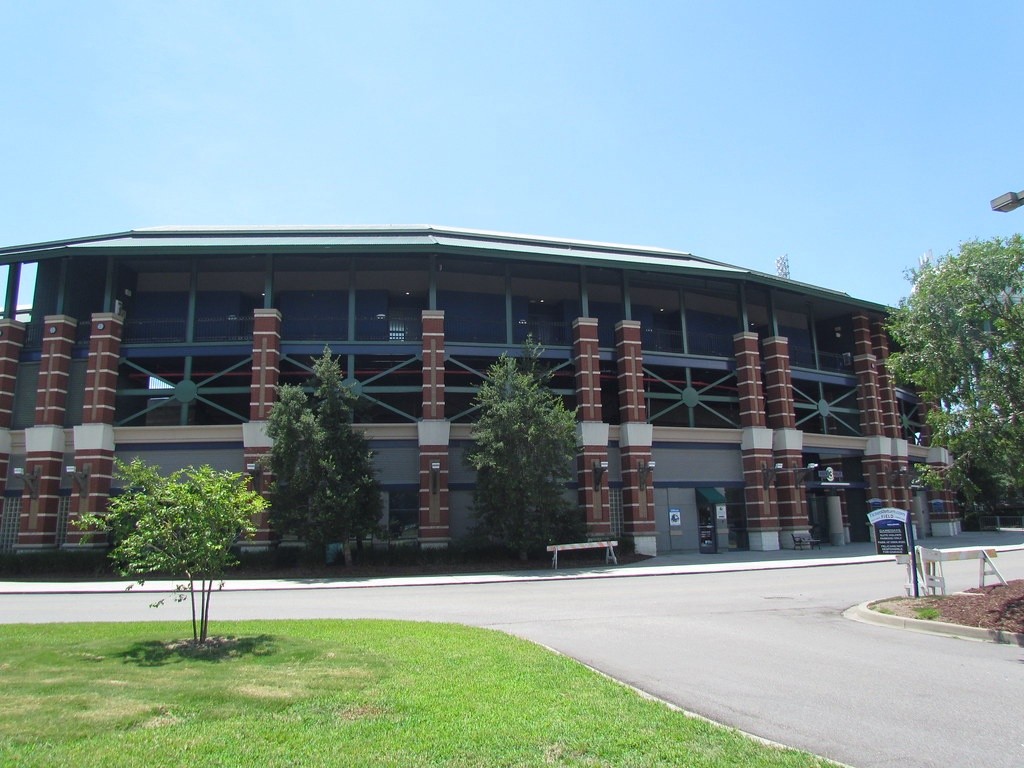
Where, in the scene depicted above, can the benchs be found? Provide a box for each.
[791,533,821,551]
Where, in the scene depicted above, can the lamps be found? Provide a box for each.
[431,463,440,495]
[593,461,608,492]
[13,467,40,500]
[66,465,90,498]
[247,463,260,494]
[638,461,655,491]
[886,465,907,489]
[794,463,818,489]
[762,463,783,491]
